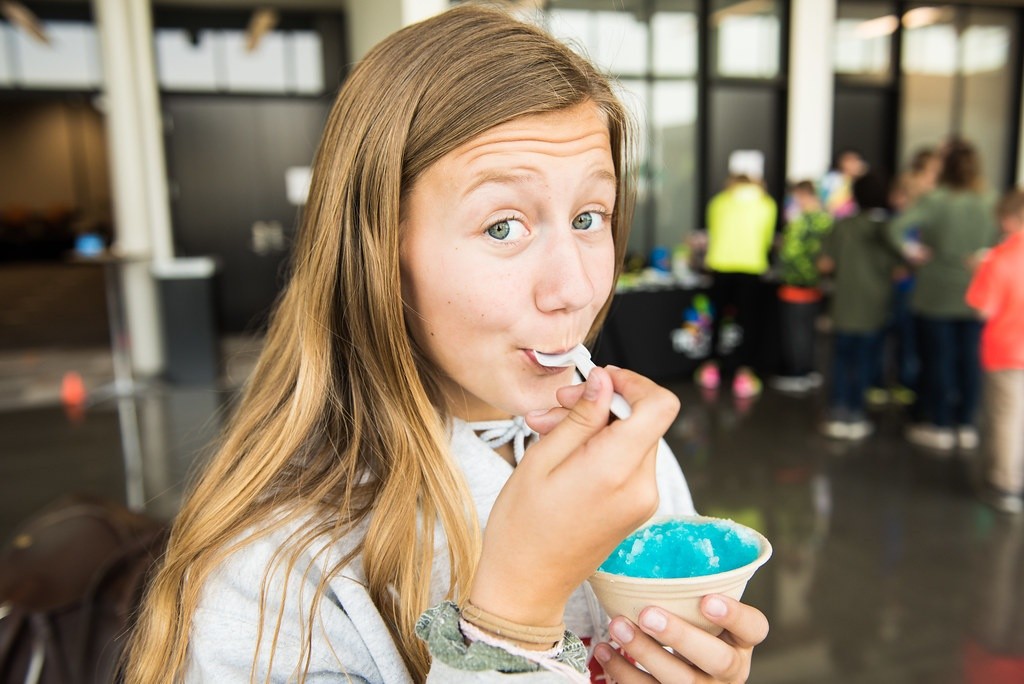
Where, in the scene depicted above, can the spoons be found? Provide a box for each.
[535,344,631,421]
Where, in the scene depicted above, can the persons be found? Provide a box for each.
[819,170,908,438]
[885,139,999,450]
[964,194,1023,514]
[696,149,779,397]
[674,228,710,287]
[124,7,768,684]
[774,151,938,408]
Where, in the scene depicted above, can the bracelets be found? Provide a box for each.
[459,601,592,684]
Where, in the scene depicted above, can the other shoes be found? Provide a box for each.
[695,367,720,388]
[906,423,977,449]
[862,386,913,406]
[821,417,872,439]
[732,377,761,396]
[771,370,825,393]
[976,482,1023,515]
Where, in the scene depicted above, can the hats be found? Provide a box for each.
[728,149,765,184]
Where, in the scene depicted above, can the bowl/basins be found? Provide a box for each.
[587,517,772,639]
